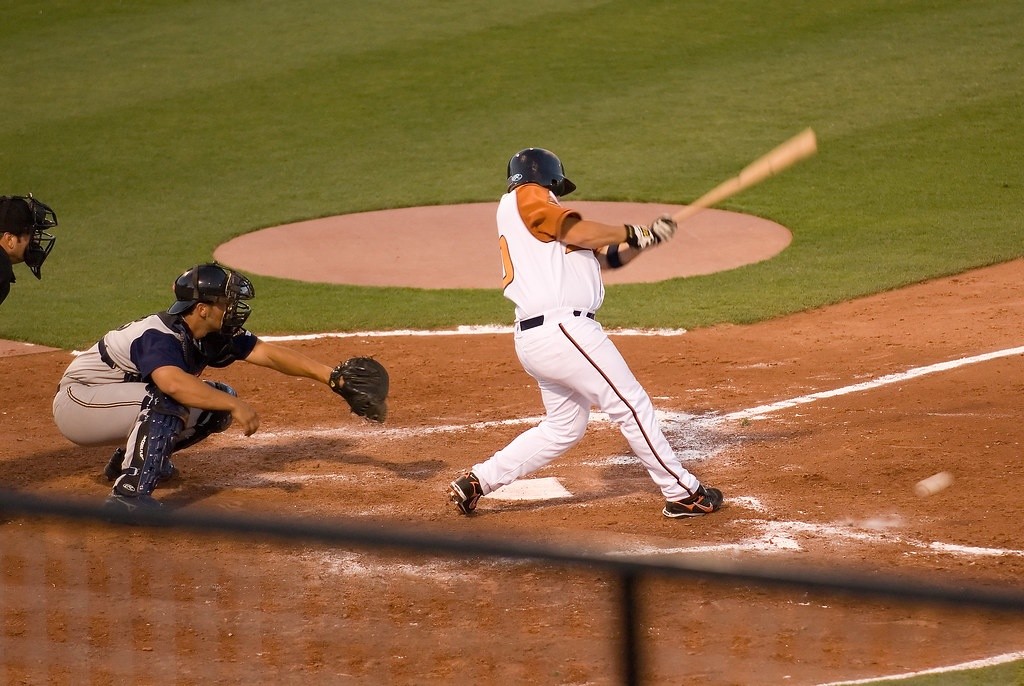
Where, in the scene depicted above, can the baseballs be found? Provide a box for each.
[912,463,961,509]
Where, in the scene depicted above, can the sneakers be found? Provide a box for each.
[105,447,174,481]
[662,485,723,518]
[102,493,168,520]
[446,471,483,515]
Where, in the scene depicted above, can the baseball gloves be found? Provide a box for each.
[327,351,390,423]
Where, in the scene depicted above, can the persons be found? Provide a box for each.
[445,148,724,519]
[52,260,390,512]
[0,195,58,305]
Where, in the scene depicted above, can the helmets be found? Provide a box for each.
[167,264,230,314]
[507,148,576,197]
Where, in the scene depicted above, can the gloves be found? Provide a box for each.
[625,224,654,249]
[651,217,678,245]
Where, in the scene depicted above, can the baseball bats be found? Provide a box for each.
[626,123,823,251]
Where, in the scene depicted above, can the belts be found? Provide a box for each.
[521,311,594,330]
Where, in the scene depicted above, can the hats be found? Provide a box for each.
[0,195,34,232]
